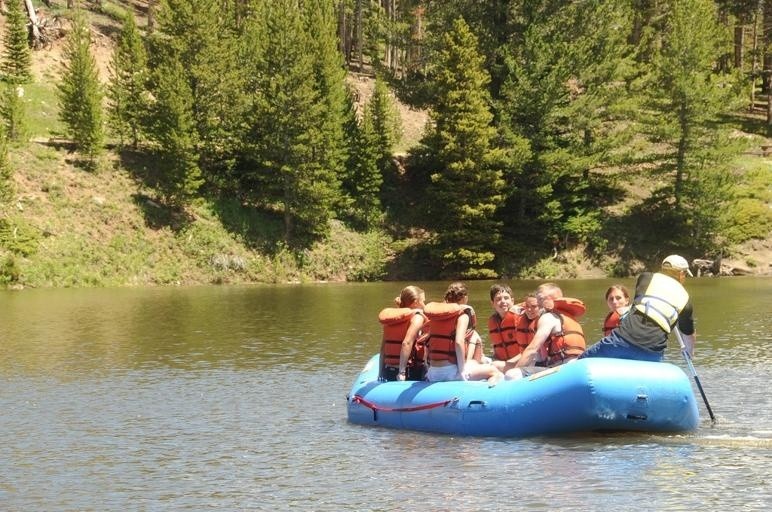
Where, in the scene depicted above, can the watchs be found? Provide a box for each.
[398,370,406,376]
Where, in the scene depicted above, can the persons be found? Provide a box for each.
[491,290,547,370]
[601,284,632,337]
[424,282,499,382]
[487,283,519,359]
[504,282,585,382]
[574,252,696,363]
[465,328,484,363]
[377,284,425,380]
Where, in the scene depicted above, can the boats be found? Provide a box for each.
[348,352,700,436]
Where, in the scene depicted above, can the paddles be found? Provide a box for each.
[675,326,714,424]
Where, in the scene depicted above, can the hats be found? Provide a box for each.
[661,253,694,279]
